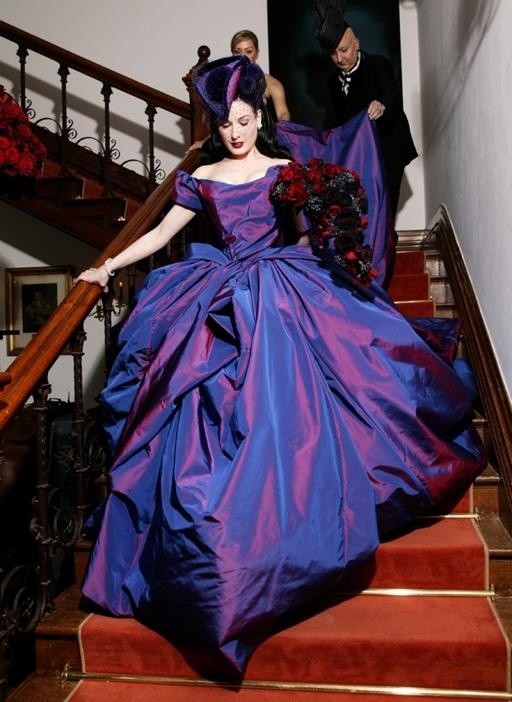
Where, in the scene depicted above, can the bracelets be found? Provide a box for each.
[105,257,114,278]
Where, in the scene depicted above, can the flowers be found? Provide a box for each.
[0,79,53,188]
[266,155,382,295]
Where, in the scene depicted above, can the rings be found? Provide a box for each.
[377,105,383,113]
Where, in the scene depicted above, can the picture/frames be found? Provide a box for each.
[3,263,77,359]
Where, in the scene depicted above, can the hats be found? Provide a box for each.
[314,12,348,58]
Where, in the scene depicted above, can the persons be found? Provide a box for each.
[73,55,489,680]
[230,30,291,123]
[306,12,419,230]
